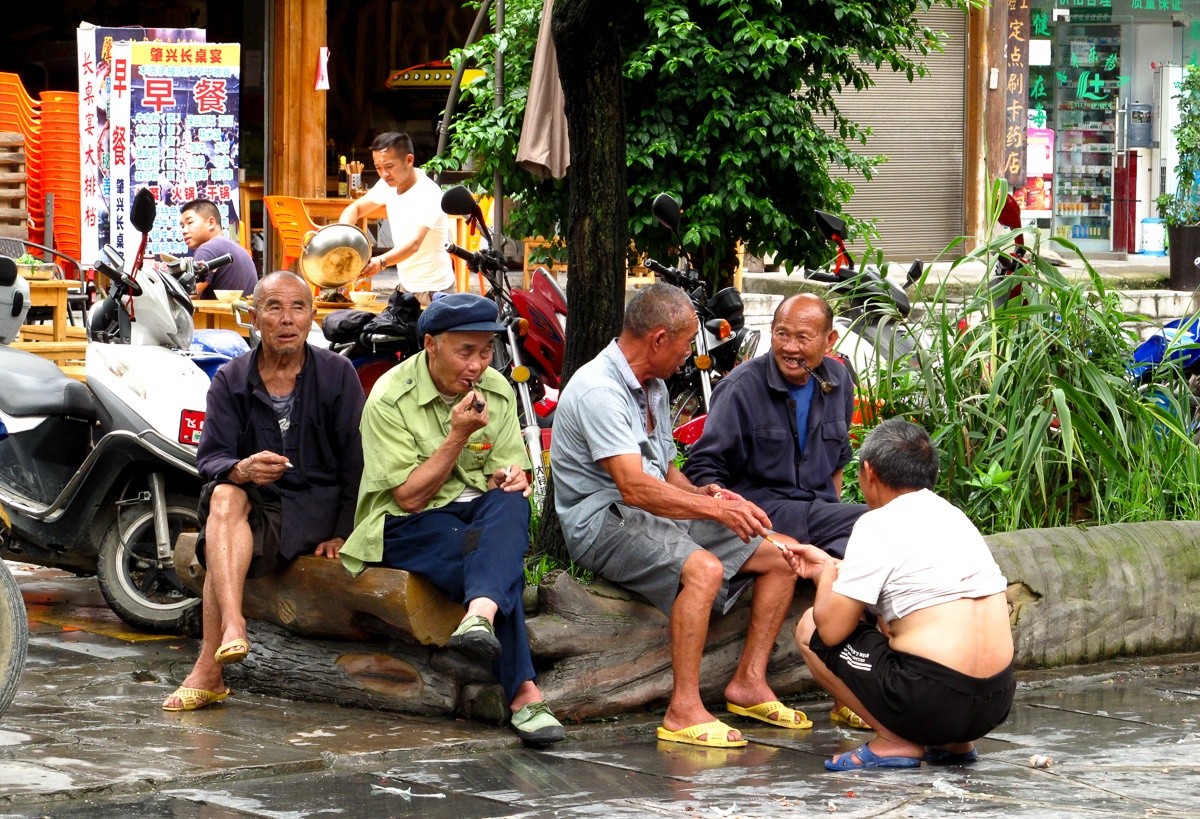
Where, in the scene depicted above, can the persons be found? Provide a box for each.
[795,419,1017,768]
[337,131,456,306]
[549,283,816,747]
[160,270,368,712]
[681,291,874,730]
[180,199,258,301]
[358,295,569,743]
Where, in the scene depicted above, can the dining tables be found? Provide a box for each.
[193,298,387,336]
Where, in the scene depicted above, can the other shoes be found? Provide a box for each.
[511,699,568,738]
[448,614,500,664]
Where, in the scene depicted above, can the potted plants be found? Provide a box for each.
[1156,63,1200,292]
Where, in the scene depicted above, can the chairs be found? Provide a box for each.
[0,71,566,376]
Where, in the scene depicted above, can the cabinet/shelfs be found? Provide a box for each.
[1051,22,1125,252]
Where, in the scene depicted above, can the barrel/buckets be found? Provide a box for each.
[1140,217,1166,256]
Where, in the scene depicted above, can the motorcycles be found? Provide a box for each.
[187,186,761,515]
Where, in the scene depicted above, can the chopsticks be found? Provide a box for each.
[346,160,364,174]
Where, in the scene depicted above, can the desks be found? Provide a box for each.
[29,279,82,367]
[239,178,340,250]
[302,195,467,291]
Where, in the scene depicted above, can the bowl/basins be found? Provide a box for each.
[16,262,56,280]
[213,289,244,302]
[349,291,378,306]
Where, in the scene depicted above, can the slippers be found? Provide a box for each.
[830,704,873,731]
[163,687,230,712]
[825,740,921,770]
[726,700,813,729]
[925,746,978,763]
[657,718,749,748]
[214,639,249,664]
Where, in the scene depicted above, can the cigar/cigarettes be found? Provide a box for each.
[283,461,294,468]
[766,536,788,551]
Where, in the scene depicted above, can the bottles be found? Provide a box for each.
[1089,223,1110,239]
[1122,104,1154,146]
[338,156,348,197]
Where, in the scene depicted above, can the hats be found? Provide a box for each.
[418,293,508,337]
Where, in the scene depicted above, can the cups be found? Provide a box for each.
[347,174,362,198]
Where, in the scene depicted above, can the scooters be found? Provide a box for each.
[805,193,1200,481]
[0,188,233,632]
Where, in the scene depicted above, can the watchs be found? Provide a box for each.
[378,254,386,271]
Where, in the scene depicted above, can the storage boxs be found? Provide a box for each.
[0,255,31,345]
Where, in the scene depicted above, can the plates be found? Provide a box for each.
[313,301,357,308]
